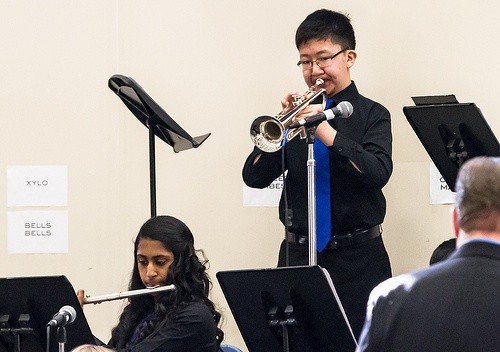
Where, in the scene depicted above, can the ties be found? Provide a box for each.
[313,98,332,253]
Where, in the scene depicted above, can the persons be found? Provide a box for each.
[69,343,115,352]
[76,215,226,352]
[241,9,393,343]
[356,155,500,352]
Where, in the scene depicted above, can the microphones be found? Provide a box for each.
[46,305,77,329]
[288,101,353,129]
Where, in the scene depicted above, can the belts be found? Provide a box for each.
[286,224,384,248]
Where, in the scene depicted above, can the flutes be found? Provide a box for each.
[81,283,177,305]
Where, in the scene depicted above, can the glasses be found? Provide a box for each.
[296,46,348,71]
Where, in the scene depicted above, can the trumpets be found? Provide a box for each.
[250,78,328,153]
